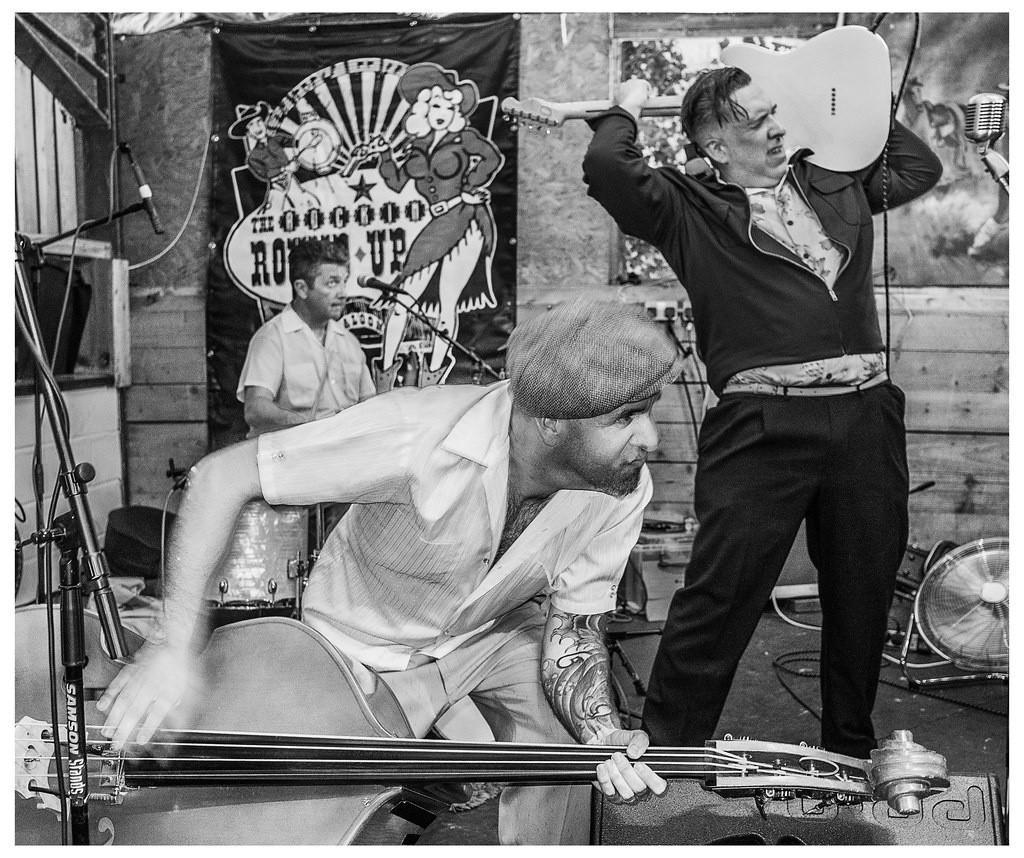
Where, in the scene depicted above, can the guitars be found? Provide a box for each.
[500,24,892,172]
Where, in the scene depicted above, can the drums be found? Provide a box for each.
[204,499,310,619]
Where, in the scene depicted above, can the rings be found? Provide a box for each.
[623,798,635,803]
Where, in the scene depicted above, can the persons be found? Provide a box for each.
[97,298,685,846]
[582,78,942,760]
[236,240,379,543]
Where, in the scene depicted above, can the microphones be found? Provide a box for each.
[126,145,165,235]
[357,273,408,296]
[965,93,1009,196]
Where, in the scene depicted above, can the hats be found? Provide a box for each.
[507,301,684,419]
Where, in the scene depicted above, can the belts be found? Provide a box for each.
[722,372,888,397]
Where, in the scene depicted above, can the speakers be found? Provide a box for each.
[592,770,1006,845]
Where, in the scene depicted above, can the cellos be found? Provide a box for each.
[16,602,951,845]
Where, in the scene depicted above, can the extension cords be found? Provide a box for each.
[769,583,820,598]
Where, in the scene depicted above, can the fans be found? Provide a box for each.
[899,537,1009,688]
